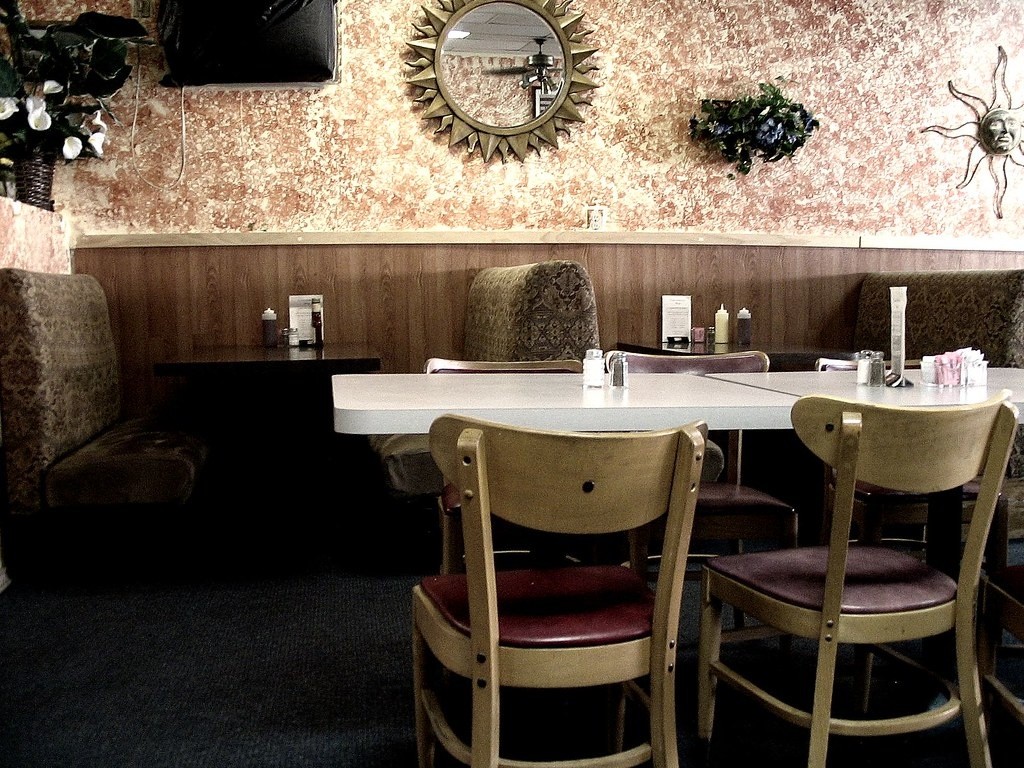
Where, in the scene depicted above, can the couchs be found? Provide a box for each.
[849,270,1024,537]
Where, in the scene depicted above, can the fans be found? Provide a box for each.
[484,36,562,76]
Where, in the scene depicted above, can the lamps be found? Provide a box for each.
[520,74,558,95]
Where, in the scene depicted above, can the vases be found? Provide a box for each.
[15,141,55,206]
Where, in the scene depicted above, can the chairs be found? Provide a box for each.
[606,347,802,663]
[393,414,711,767]
[701,389,1016,768]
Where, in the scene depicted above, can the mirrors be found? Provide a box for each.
[404,0,602,162]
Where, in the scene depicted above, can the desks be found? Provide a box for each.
[620,337,841,485]
[163,344,380,586]
[330,364,1024,681]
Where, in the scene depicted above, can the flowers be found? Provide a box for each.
[684,86,821,171]
[0,0,148,165]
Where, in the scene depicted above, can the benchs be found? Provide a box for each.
[361,254,725,566]
[0,269,207,590]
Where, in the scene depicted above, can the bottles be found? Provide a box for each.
[311,297,323,350]
[736,307,752,346]
[856,350,875,384]
[706,326,715,343]
[582,349,605,388]
[869,352,886,387]
[715,303,729,354]
[262,307,277,347]
